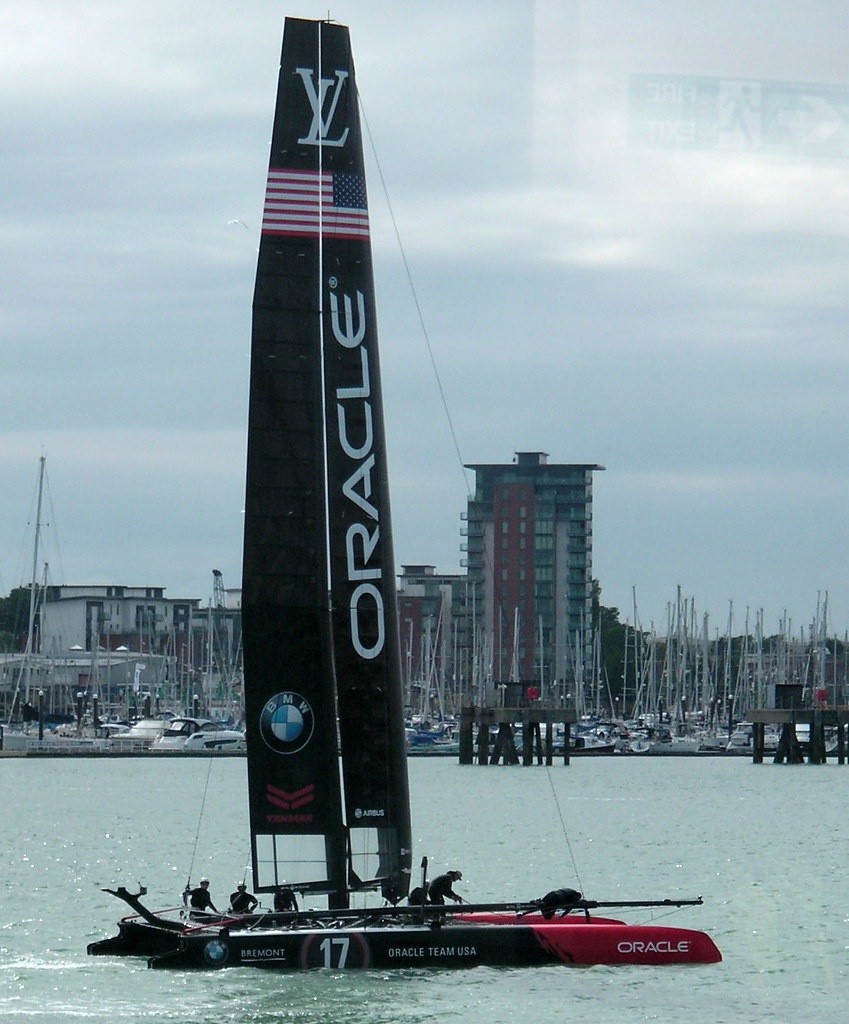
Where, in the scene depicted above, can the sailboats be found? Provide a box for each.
[88,13,723,973]
[0,455,849,756]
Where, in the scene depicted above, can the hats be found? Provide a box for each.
[454,871,462,880]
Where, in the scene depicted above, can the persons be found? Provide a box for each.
[408,879,433,906]
[183,877,222,924]
[230,880,258,915]
[428,870,462,905]
[273,880,300,913]
